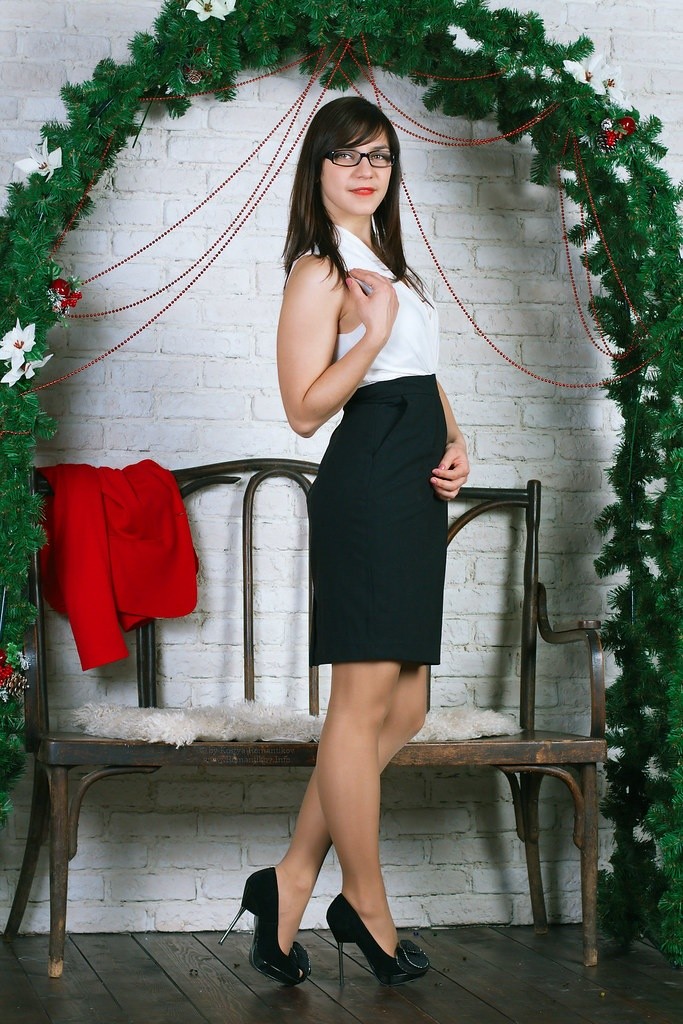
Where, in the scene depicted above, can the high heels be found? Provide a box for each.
[326,893,430,987]
[218,868,311,986]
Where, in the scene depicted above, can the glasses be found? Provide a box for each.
[324,150,395,168]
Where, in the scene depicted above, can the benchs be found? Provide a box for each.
[0,458,608,979]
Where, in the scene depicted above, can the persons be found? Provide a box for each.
[219,97,470,987]
